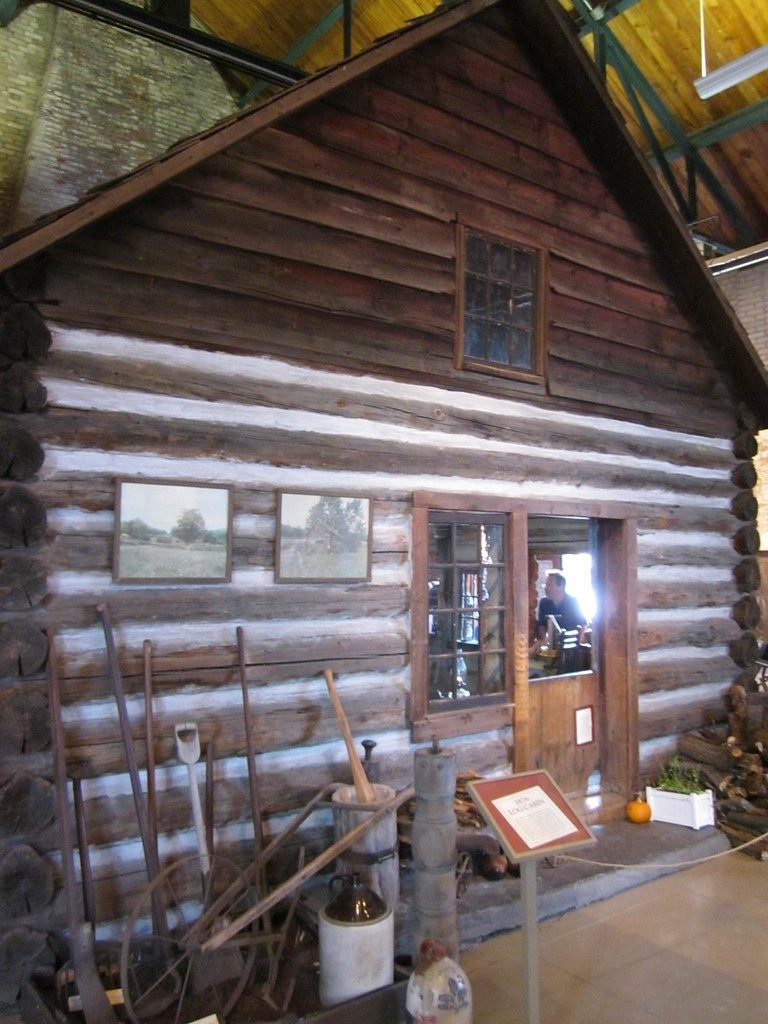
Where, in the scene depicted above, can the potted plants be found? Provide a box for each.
[645,761,717,832]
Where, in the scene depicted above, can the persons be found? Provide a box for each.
[528,570,591,657]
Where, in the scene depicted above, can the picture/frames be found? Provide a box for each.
[107,475,235,586]
[271,489,377,588]
